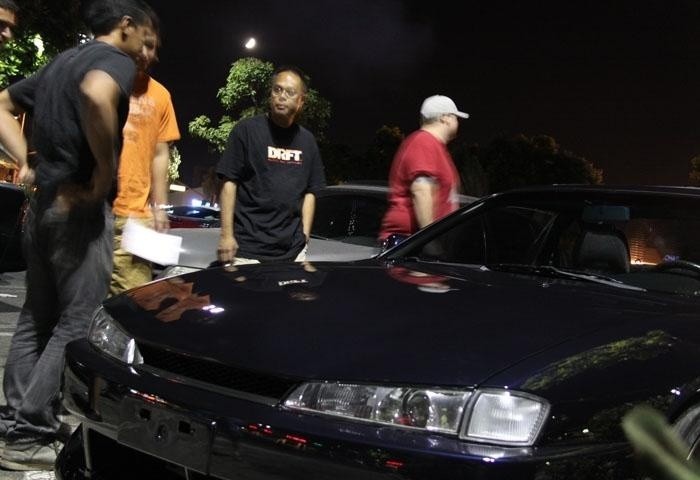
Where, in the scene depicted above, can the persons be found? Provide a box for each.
[111,2,180,299]
[376,94,471,257]
[1,0,150,472]
[0,0,17,49]
[216,68,327,266]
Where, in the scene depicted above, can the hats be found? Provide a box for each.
[420,94,470,121]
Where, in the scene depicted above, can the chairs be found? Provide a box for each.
[570,230,630,283]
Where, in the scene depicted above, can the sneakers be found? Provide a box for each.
[0,438,65,472]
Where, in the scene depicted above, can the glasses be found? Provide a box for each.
[268,86,298,99]
